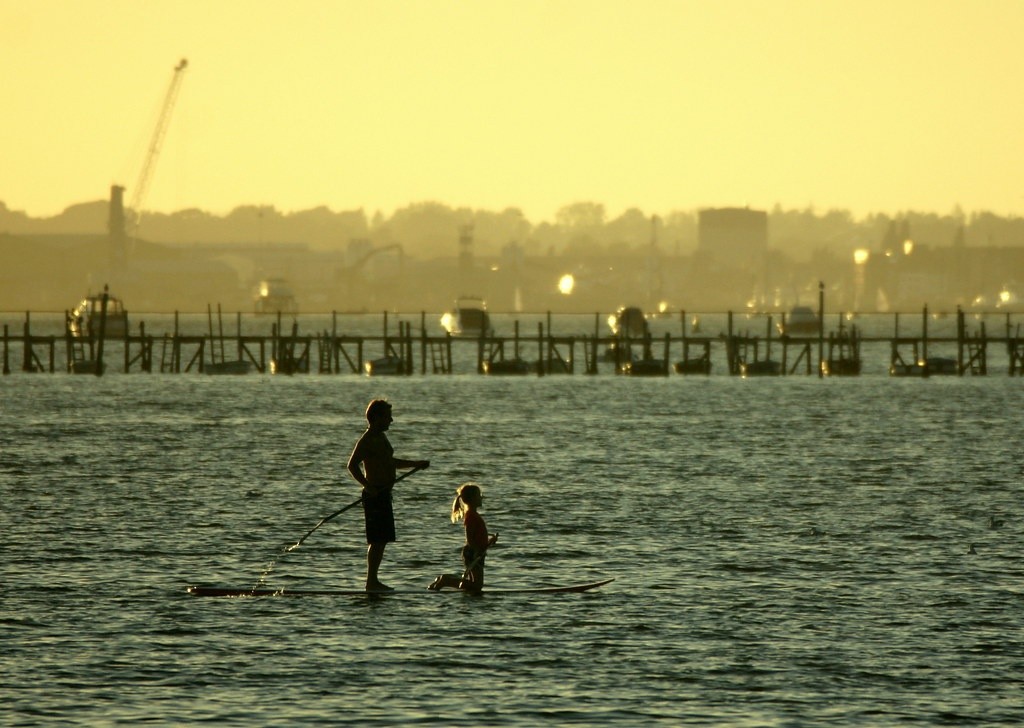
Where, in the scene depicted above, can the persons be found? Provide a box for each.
[346,398,431,594]
[427,484,499,591]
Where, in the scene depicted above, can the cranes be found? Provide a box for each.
[121,56,190,237]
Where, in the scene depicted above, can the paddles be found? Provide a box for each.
[456,532,500,593]
[295,460,431,548]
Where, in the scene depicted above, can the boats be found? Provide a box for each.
[189,578,617,597]
[607,305,649,339]
[440,295,492,337]
[66,293,131,337]
[786,306,819,335]
